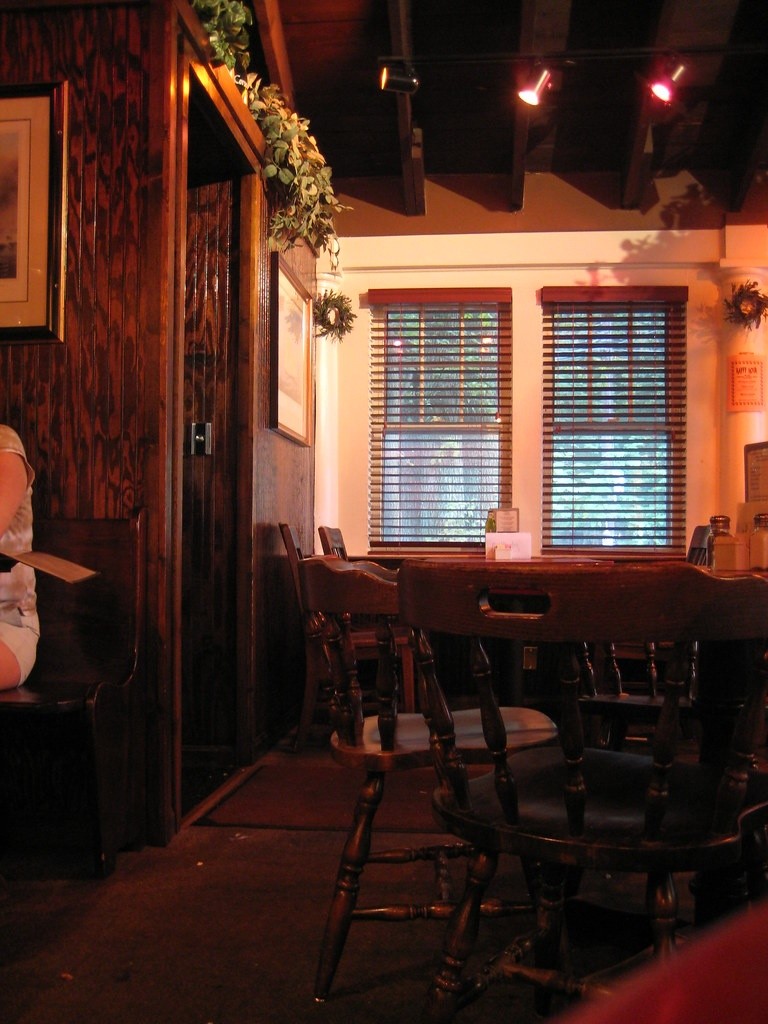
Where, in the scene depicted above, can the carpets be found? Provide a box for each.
[189,763,453,834]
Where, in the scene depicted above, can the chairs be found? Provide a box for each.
[575,641,698,752]
[0,517,145,882]
[685,525,711,565]
[306,559,557,1002]
[318,525,348,561]
[393,559,768,1024]
[280,523,414,753]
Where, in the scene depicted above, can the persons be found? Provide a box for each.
[0,423,41,691]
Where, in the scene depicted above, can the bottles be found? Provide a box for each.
[706,514,738,575]
[745,512,768,578]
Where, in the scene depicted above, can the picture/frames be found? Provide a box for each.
[271,250,313,448]
[0,79,69,346]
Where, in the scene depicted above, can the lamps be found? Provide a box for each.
[650,59,688,102]
[379,64,419,94]
[519,65,552,105]
